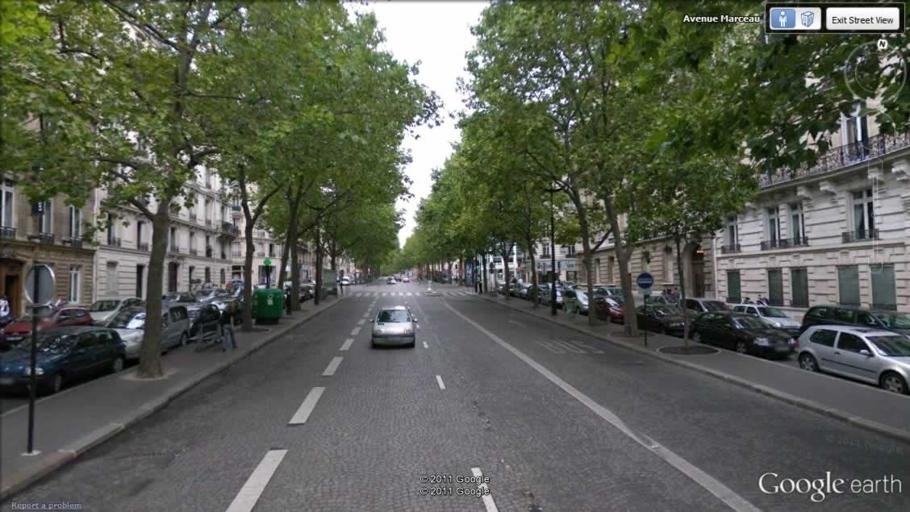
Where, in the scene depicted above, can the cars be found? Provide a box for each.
[497,269,909,399]
[368,304,419,347]
[1,275,316,405]
[378,270,414,286]
[334,272,357,286]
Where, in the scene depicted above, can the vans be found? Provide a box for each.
[431,268,450,284]
